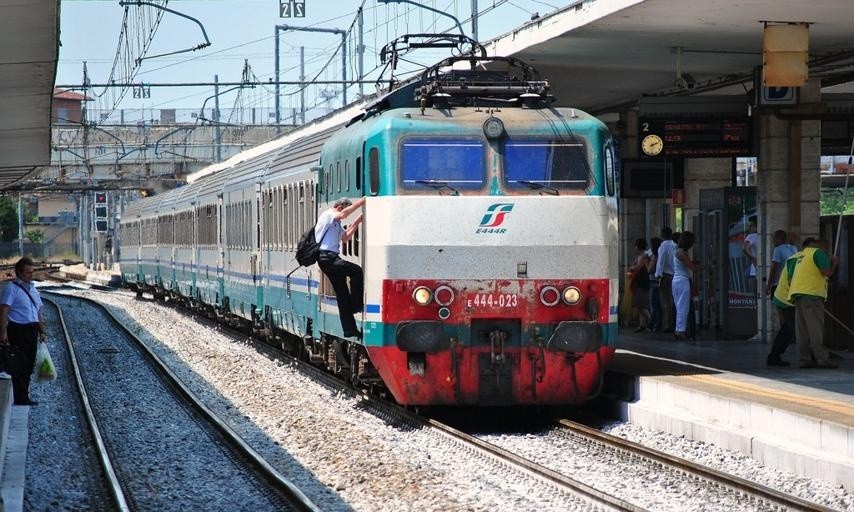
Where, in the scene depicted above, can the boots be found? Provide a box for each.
[766,335,793,367]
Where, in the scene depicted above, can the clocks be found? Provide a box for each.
[641,134,664,156]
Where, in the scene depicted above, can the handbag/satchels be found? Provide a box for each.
[0,339,20,375]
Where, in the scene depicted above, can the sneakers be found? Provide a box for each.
[799,359,817,368]
[817,359,838,368]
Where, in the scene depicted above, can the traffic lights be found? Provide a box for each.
[95,191,107,205]
[95,220,108,233]
[94,207,107,218]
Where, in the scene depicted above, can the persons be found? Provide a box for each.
[625,227,695,341]
[765,230,839,369]
[296,196,365,337]
[0,348,11,379]
[0,256,47,406]
[742,215,758,341]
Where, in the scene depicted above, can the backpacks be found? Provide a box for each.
[295,226,320,267]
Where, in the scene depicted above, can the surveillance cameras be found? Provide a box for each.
[681,74,696,90]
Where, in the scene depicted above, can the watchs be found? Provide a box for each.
[39,330,46,336]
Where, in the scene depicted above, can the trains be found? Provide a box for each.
[113,79,624,417]
